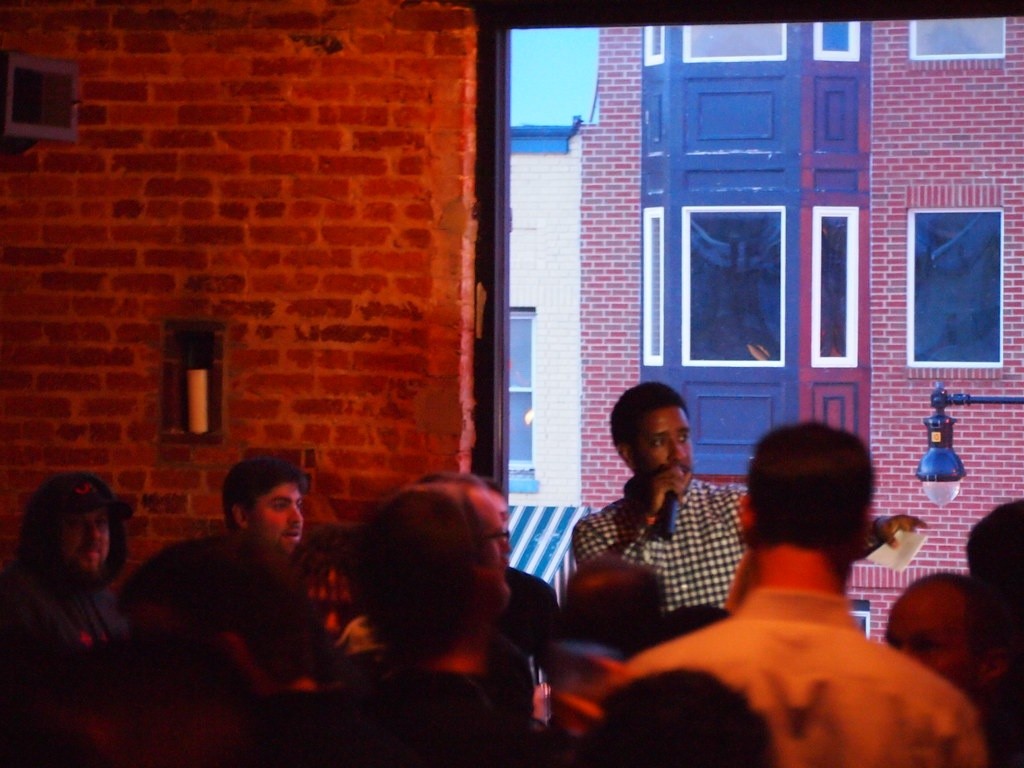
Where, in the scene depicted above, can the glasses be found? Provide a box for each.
[479,530,510,544]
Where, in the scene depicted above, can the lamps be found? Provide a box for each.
[914,380,1024,506]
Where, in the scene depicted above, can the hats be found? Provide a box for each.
[45,474,133,521]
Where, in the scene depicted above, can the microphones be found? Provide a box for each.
[656,490,679,540]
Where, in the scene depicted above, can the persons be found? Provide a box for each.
[0,424,1024,768]
[572,382,931,614]
